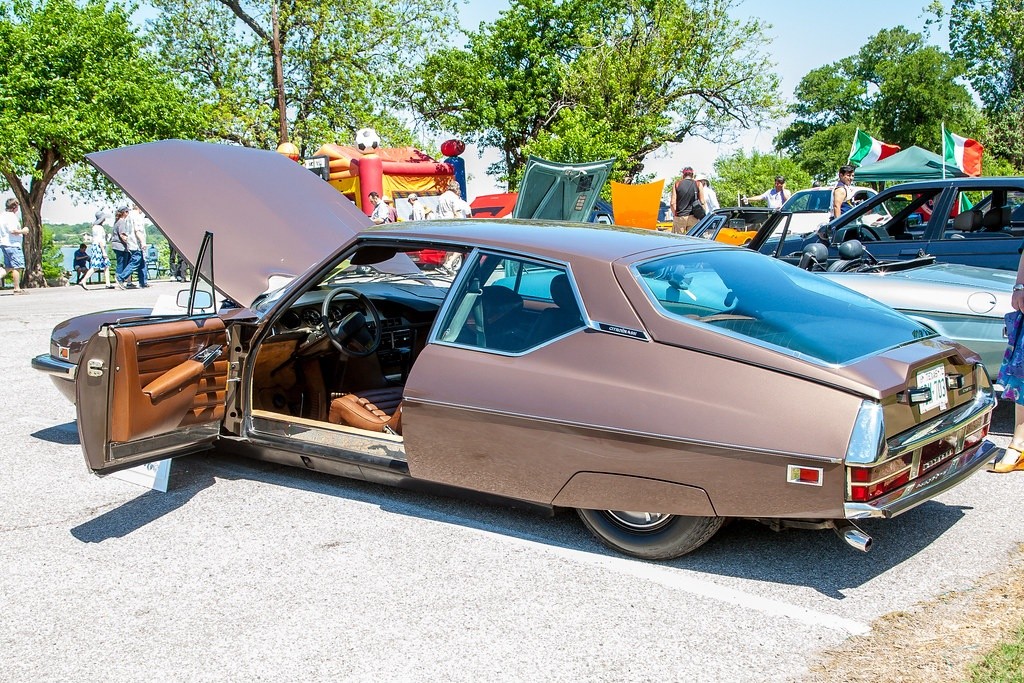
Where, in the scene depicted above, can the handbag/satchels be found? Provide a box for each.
[692,201,706,220]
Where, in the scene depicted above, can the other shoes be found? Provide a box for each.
[140,284,151,287]
[14,289,29,295]
[126,284,137,288]
[115,277,125,291]
[79,282,88,291]
[106,285,115,289]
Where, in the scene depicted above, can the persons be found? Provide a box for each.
[986,241,1024,473]
[700,178,720,228]
[670,167,705,235]
[368,191,392,281]
[74,244,91,283]
[78,211,116,290]
[0,199,30,296]
[382,195,398,222]
[741,175,791,218]
[169,243,187,283]
[408,193,425,220]
[111,206,140,289]
[114,205,152,290]
[829,166,856,225]
[435,181,472,275]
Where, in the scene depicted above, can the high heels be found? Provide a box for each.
[988,446,1024,473]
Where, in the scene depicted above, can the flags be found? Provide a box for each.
[944,127,983,177]
[849,130,901,167]
[961,192,973,212]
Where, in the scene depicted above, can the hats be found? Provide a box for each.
[118,206,131,213]
[695,175,710,187]
[94,211,111,225]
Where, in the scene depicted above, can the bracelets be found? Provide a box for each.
[1013,284,1023,291]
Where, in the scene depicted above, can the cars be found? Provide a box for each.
[32,140,1024,561]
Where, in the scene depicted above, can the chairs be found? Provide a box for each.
[798,243,828,271]
[827,239,863,272]
[328,386,404,436]
[981,206,1011,232]
[953,211,984,231]
[523,272,579,349]
[474,285,524,349]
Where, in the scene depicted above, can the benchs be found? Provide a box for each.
[73,268,171,284]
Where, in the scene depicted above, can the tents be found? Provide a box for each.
[853,145,955,193]
